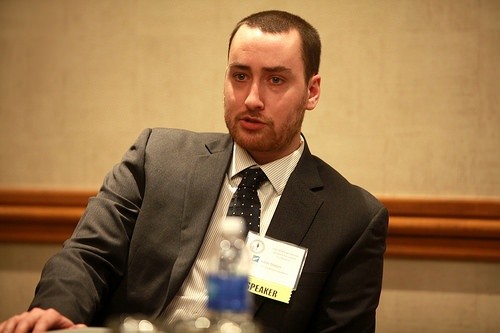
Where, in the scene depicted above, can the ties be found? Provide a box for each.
[220,167,268,275]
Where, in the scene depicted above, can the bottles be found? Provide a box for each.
[207,216,249,333]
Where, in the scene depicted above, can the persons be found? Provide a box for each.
[0,10,389,333]
